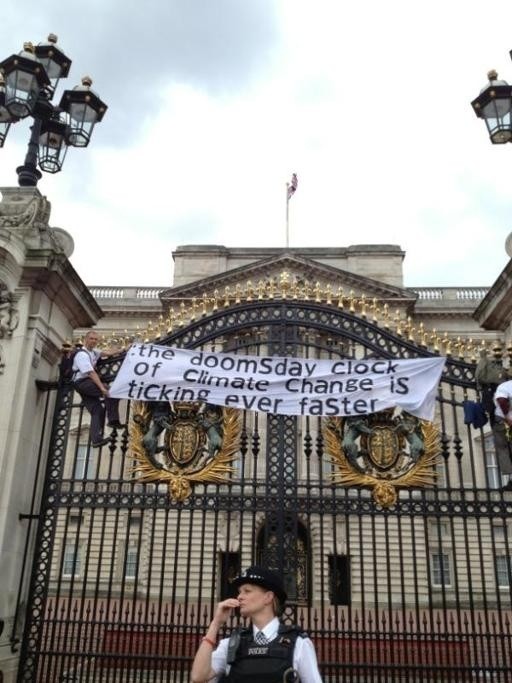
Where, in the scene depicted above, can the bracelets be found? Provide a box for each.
[201,637,217,650]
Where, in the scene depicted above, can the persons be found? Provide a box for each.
[72,330,135,448]
[493,379,512,490]
[188,565,323,683]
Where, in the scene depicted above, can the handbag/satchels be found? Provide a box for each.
[475,358,506,385]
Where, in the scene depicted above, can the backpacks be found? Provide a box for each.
[59,349,96,385]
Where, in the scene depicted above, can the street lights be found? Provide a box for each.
[0,31,109,187]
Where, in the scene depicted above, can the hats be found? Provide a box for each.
[233,566,287,605]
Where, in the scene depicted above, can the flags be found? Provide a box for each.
[287,172,297,199]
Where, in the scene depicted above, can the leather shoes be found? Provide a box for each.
[93,437,110,448]
[107,421,122,429]
